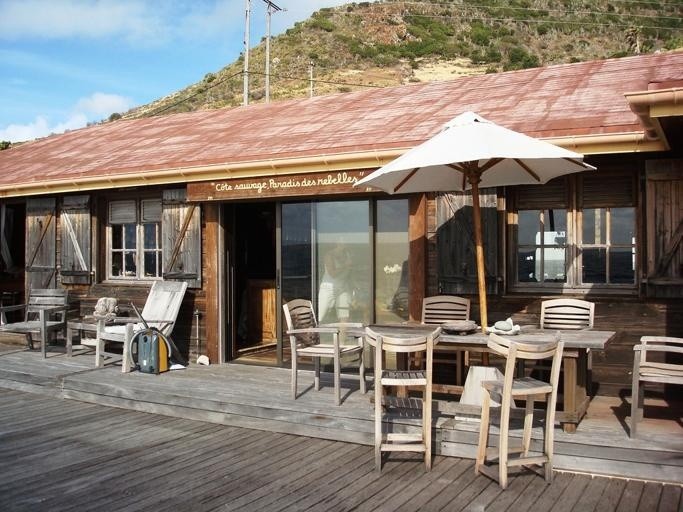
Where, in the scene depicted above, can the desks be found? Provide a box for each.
[65,314,115,357]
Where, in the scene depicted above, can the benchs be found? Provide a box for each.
[0,285,74,361]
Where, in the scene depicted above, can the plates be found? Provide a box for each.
[442,320,477,334]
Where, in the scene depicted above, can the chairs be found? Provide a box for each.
[95,275,190,375]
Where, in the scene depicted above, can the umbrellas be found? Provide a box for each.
[348,109,600,368]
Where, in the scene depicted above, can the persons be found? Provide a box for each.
[386,278,408,323]
[318,236,358,346]
